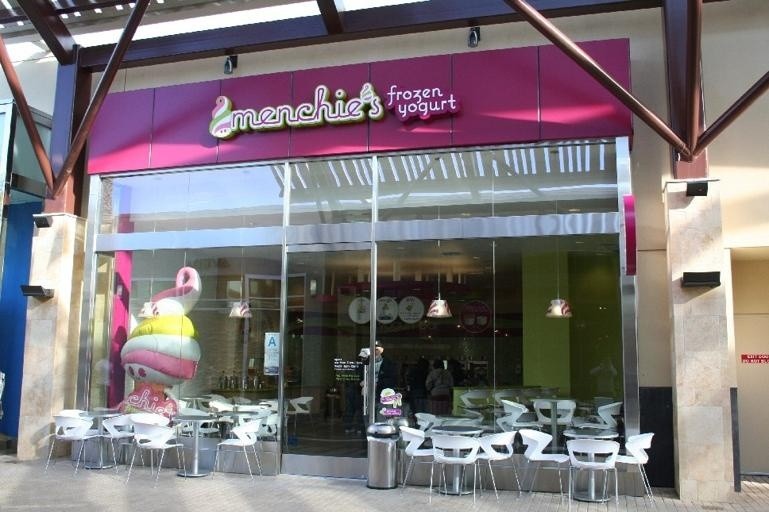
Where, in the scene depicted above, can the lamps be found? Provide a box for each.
[546,199,573,319]
[426,207,452,319]
[228,216,253,318]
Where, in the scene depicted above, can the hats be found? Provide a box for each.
[359,349,370,358]
[375,341,382,346]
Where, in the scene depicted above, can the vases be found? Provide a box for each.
[386,417,395,425]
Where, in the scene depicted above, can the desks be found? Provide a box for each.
[433,424,483,494]
[86,410,120,469]
[216,404,271,439]
[563,430,619,503]
[174,415,218,477]
[179,395,212,437]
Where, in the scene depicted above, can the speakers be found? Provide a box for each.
[686,182,709,196]
[35,216,51,227]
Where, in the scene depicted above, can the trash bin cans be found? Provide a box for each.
[366,423,399,489]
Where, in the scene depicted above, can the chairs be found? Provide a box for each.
[501,401,542,431]
[257,414,278,440]
[45,409,97,472]
[215,420,263,479]
[604,431,653,503]
[137,222,160,320]
[415,413,437,437]
[566,438,621,503]
[574,401,622,431]
[126,413,188,485]
[287,397,318,438]
[519,428,569,497]
[477,431,523,499]
[233,395,251,403]
[496,390,528,414]
[183,409,218,438]
[429,434,478,502]
[535,400,582,457]
[97,414,145,474]
[457,390,489,409]
[399,426,447,495]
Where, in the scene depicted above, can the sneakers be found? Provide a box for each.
[345,428,356,433]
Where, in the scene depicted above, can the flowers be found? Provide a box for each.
[378,388,403,418]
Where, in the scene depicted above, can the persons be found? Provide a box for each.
[425,359,452,416]
[343,382,362,433]
[447,359,465,385]
[358,341,396,429]
